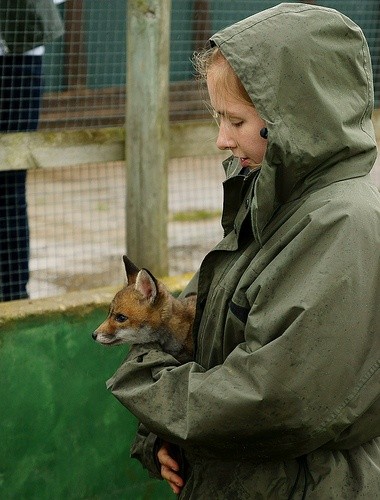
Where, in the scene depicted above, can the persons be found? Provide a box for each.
[1,0,66,302]
[106,3,380,500]
